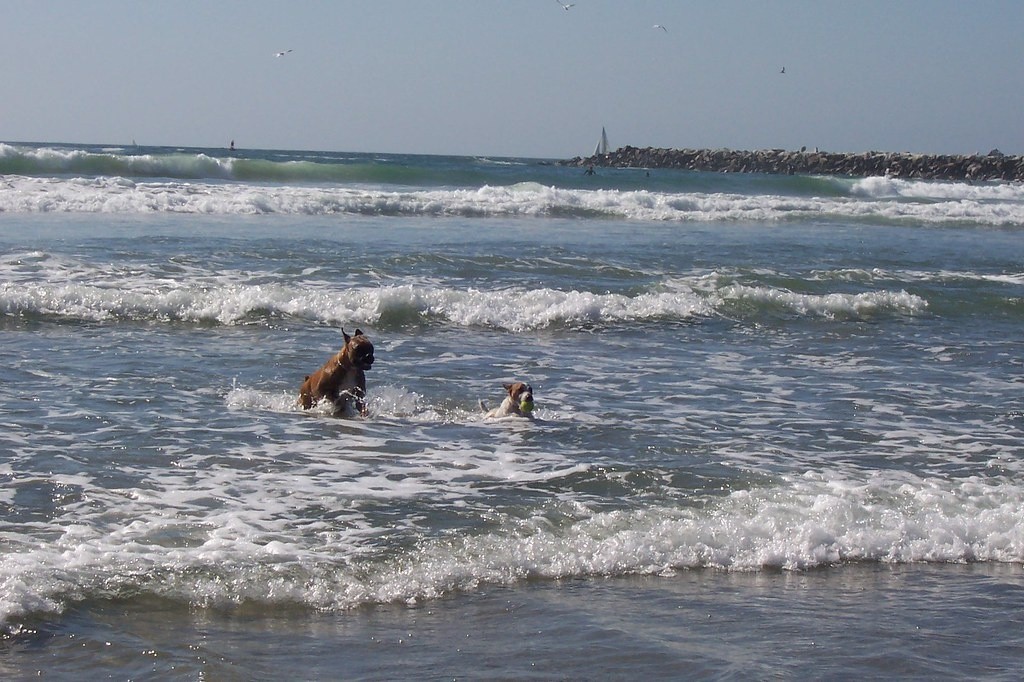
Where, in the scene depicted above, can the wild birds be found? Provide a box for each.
[275,48,293,58]
[652,24,669,32]
[555,1,576,11]
[775,65,787,73]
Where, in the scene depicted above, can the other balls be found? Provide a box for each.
[520,401,534,413]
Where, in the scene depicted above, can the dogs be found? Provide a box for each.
[294,326,375,418]
[477,379,537,424]
